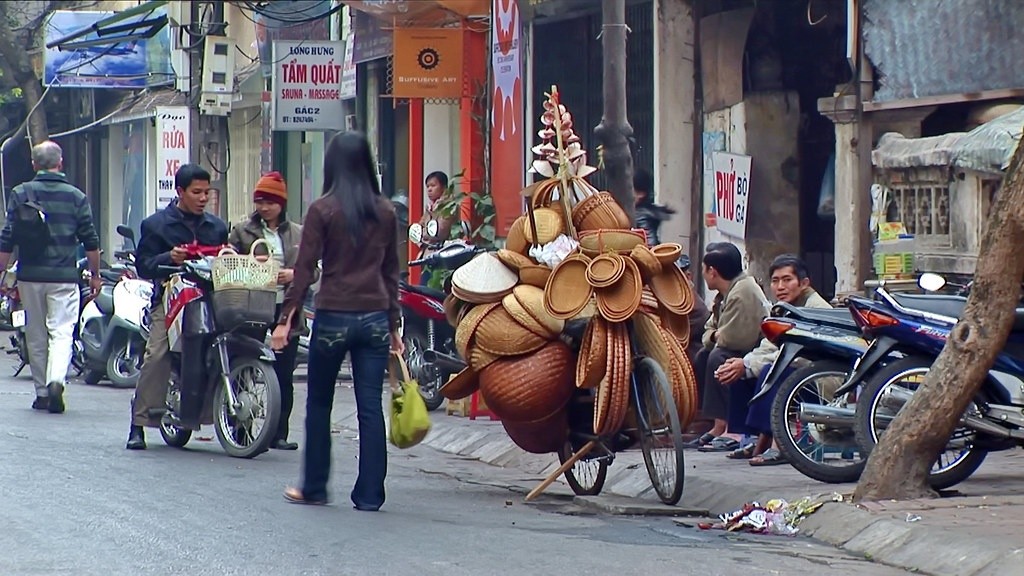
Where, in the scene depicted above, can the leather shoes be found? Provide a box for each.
[126,431,146,449]
[271,439,298,449]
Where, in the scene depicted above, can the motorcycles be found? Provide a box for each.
[389,213,498,413]
[291,304,358,381]
[747,270,974,485]
[149,238,282,462]
[0,271,32,378]
[79,222,153,388]
[845,280,1024,489]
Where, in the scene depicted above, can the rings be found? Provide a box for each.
[275,343,279,347]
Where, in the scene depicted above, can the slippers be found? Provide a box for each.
[698,437,740,452]
[688,432,714,449]
[749,448,789,466]
[726,443,768,459]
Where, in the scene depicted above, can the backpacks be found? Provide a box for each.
[13,182,49,252]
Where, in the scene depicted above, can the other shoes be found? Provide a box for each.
[282,487,327,505]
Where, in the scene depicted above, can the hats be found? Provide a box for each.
[253,172,287,208]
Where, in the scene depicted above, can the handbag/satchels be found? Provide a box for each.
[389,351,430,449]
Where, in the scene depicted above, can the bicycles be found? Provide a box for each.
[561,317,685,508]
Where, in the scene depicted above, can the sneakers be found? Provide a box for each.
[47,382,65,413]
[32,395,48,409]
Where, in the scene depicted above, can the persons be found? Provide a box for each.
[0,140,102,414]
[675,242,870,466]
[633,169,677,250]
[273,130,405,511]
[127,163,320,450]
[414,171,460,386]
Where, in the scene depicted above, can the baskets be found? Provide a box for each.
[444,175,697,454]
[209,238,280,330]
